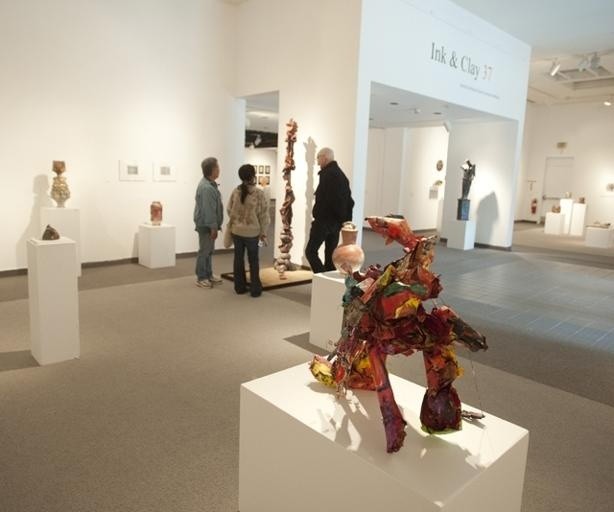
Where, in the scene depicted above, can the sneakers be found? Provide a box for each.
[196,276,225,290]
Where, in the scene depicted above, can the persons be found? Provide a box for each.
[303,147,355,273]
[192,156,225,291]
[226,163,270,297]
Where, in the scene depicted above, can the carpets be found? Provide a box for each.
[512,224,614,259]
[0,272,614,512]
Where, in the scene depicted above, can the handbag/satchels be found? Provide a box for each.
[223,220,233,249]
